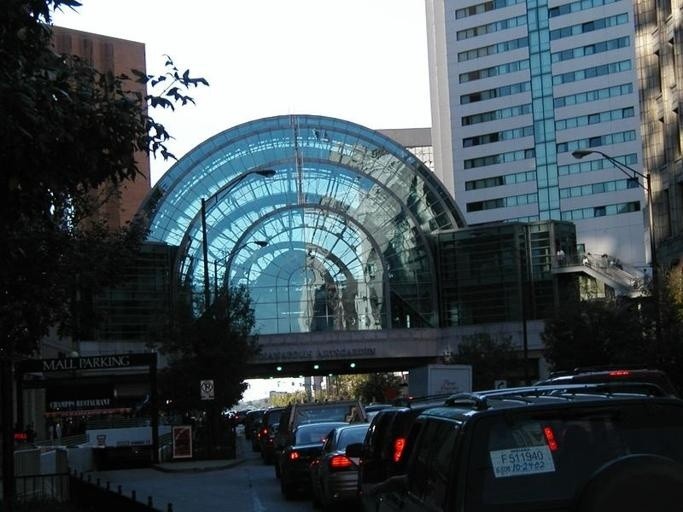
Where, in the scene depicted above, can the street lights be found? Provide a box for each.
[200,169,278,308]
[214,241,267,300]
[571,150,666,365]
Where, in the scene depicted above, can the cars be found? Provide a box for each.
[227,398,395,509]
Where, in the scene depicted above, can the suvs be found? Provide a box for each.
[358,392,457,511]
[378,380,682,511]
[530,368,677,396]
